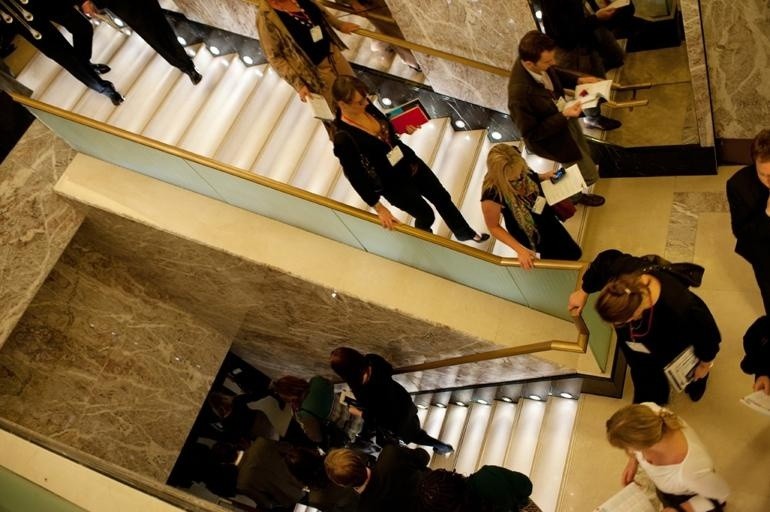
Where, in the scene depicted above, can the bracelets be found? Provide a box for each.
[700,361,715,370]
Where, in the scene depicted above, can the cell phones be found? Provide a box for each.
[550,168,566,184]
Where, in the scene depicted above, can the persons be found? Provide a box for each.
[89,0,202,86]
[603,400,732,511]
[725,128,769,317]
[328,72,491,244]
[563,247,721,407]
[0,0,125,107]
[167,348,533,512]
[500,31,606,209]
[584,0,636,70]
[478,143,583,271]
[257,1,361,151]
[530,0,625,133]
[738,311,769,395]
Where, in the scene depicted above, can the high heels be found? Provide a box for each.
[433,445,454,455]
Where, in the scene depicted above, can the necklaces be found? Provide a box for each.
[275,1,313,30]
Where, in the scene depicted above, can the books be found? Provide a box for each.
[390,106,429,138]
[305,91,335,124]
[385,98,431,133]
[594,1,632,16]
[740,388,769,418]
[596,481,656,512]
[664,346,706,394]
[567,79,616,111]
[540,163,588,206]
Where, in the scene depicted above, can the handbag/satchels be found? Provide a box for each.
[647,252,703,288]
[552,199,577,223]
[300,408,347,452]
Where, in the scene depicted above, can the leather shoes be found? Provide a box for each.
[185,69,202,85]
[90,62,111,74]
[472,231,490,244]
[109,91,124,106]
[583,115,622,131]
[574,192,605,207]
[687,374,709,402]
